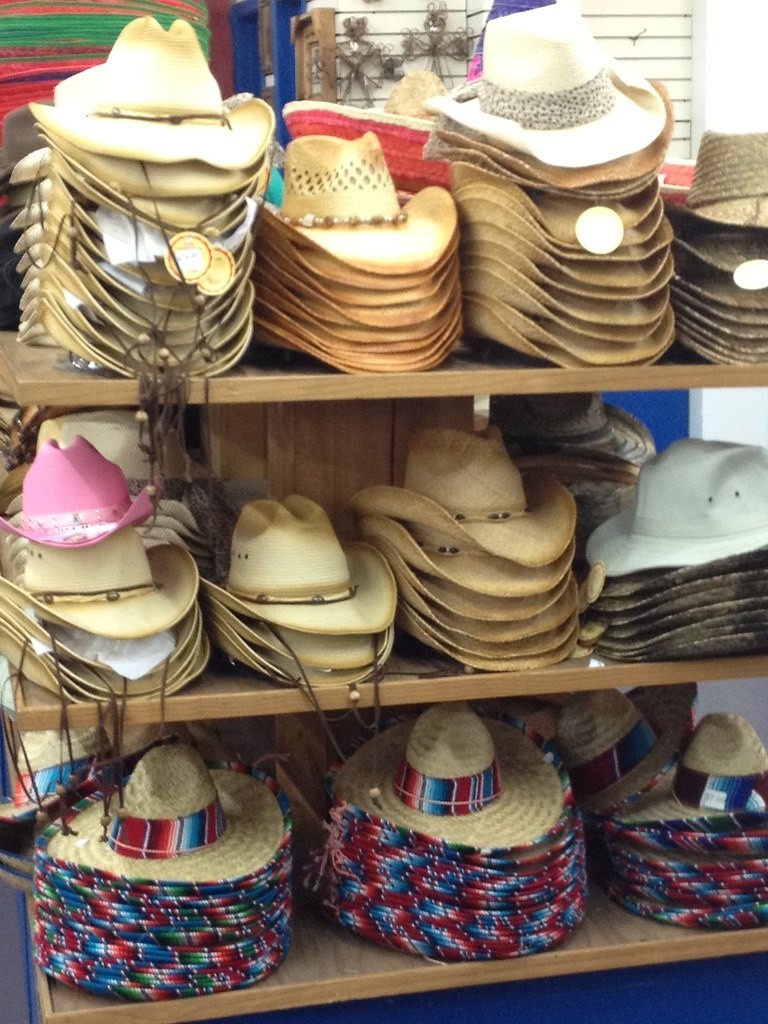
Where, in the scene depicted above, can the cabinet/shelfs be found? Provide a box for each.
[0,331,768,1024]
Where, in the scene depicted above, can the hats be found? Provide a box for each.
[0,0,768,1001]
